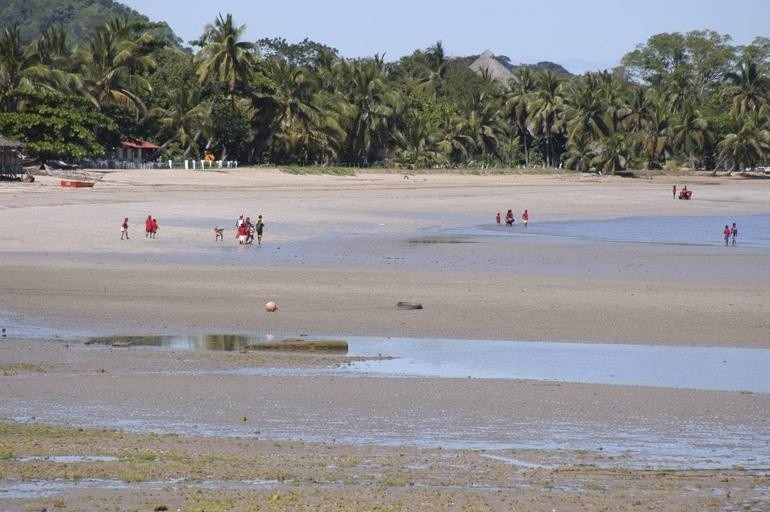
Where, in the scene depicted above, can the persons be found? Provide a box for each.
[495,212,502,224]
[504,209,515,228]
[144,213,267,248]
[730,222,738,245]
[521,209,529,227]
[119,216,132,241]
[672,184,678,199]
[723,224,731,247]
